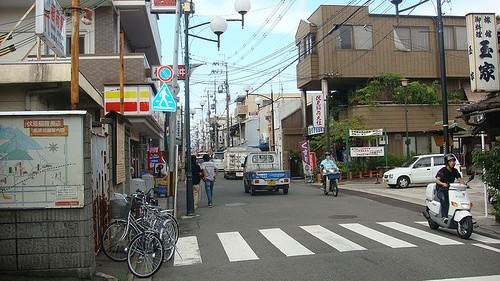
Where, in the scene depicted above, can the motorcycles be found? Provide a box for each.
[422,176,473,240]
[320,167,339,197]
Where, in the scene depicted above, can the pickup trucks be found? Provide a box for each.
[210,152,224,171]
[240,151,290,194]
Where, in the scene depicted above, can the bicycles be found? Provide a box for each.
[127,201,176,278]
[130,187,179,242]
[101,195,179,262]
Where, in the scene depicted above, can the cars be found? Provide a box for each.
[382,153,463,189]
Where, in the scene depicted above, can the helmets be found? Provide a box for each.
[444,154,456,164]
[324,152,331,156]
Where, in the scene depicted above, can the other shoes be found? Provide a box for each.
[209,204,212,208]
[473,220,477,225]
[443,218,448,224]
[321,187,325,191]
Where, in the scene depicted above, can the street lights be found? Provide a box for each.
[398,78,411,162]
[182,0,252,216]
[188,59,339,156]
[475,113,489,217]
[391,0,452,154]
[242,82,276,151]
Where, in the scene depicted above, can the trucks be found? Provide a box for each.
[223,147,261,180]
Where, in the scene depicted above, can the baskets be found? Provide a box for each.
[328,173,340,179]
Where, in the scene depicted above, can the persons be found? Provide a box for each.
[185,155,203,210]
[199,154,218,208]
[434,154,466,224]
[319,151,340,191]
